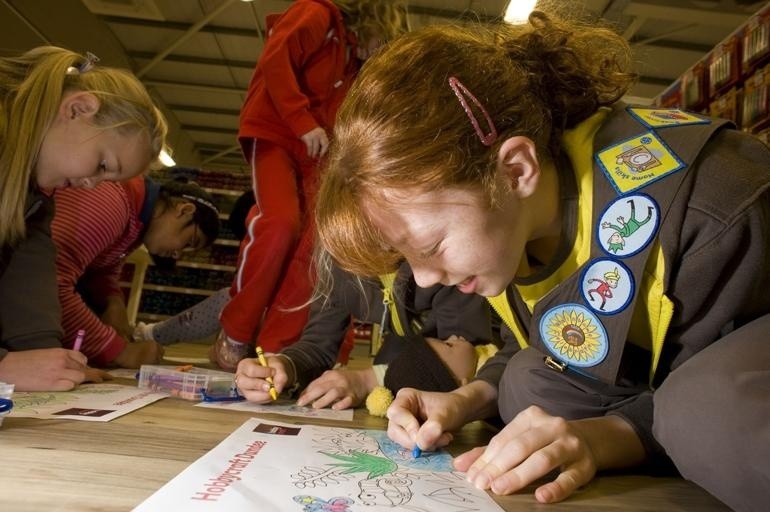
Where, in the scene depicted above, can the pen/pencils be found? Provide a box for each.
[74,330,86,350]
[136,365,231,400]
[413,445,421,458]
[256,346,277,401]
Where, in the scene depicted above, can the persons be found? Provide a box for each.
[231,244,499,420]
[0,44,171,398]
[314,8,770,510]
[131,185,257,349]
[362,328,498,420]
[44,161,221,369]
[206,0,407,380]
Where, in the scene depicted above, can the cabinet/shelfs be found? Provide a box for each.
[117,187,244,327]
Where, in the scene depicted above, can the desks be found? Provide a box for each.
[0,341,735,511]
